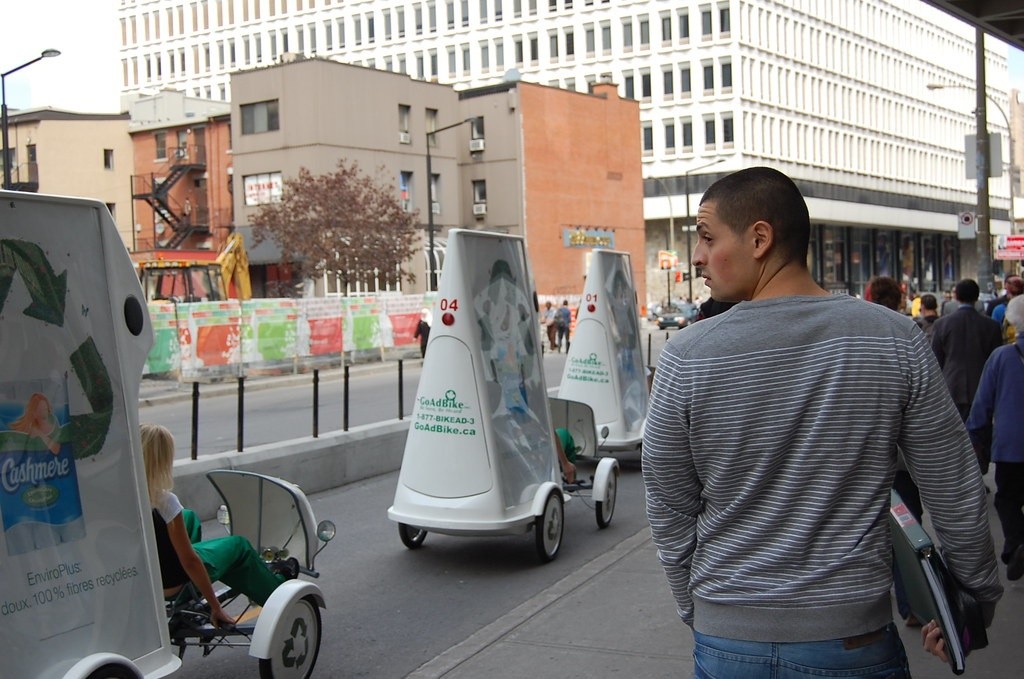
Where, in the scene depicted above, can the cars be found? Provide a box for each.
[655,302,701,331]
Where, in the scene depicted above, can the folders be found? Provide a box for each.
[889,489,966,676]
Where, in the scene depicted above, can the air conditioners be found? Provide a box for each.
[432,202,440,213]
[469,138,485,152]
[400,132,409,143]
[473,203,487,216]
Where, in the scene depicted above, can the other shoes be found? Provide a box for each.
[1007,545,1024,581]
[905,614,919,627]
[281,558,300,580]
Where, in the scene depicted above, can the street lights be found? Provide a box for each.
[0,48,63,191]
[684,157,728,303]
[925,81,1017,240]
[426,115,478,292]
[642,174,673,308]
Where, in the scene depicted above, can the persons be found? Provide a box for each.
[856,276,1024,329]
[641,168,1004,679]
[544,299,571,354]
[929,279,1002,494]
[414,309,433,359]
[139,424,300,629]
[965,294,1024,580]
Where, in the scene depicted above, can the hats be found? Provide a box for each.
[1005,277,1024,292]
